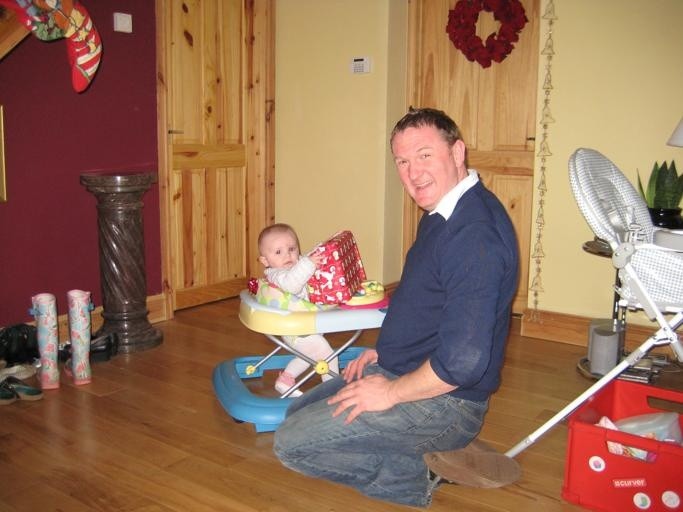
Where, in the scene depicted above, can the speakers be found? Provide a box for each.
[587,319,613,361]
[590,329,619,376]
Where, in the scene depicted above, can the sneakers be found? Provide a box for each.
[274,371,302,397]
[422,449,520,490]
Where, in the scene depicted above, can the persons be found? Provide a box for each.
[272,106,524,508]
[256,223,342,401]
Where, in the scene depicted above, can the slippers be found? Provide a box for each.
[4,375,43,401]
[0,383,16,405]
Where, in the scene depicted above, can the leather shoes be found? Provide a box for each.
[63,334,111,362]
[108,332,118,357]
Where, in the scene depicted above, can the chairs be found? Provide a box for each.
[568,147,683,384]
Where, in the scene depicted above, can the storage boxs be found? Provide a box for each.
[560,377,682,509]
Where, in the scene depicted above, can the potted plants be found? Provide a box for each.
[634,160,682,231]
